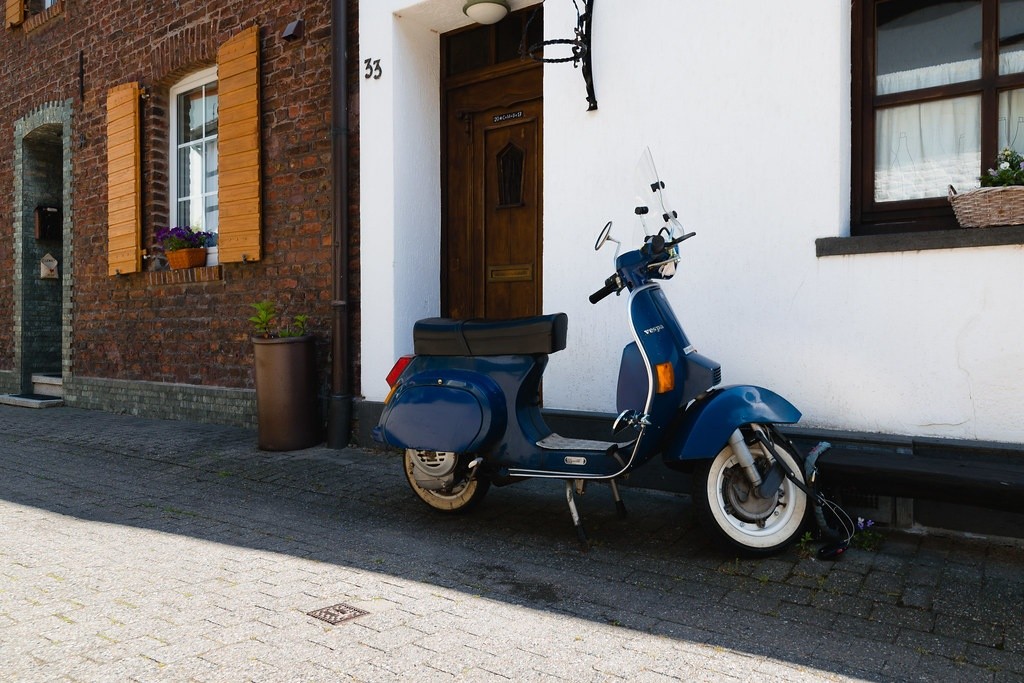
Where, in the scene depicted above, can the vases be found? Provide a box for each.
[165,248,207,270]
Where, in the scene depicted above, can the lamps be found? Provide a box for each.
[462,0,511,24]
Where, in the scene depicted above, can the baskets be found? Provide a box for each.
[165,248,207,270]
[947,183,1024,228]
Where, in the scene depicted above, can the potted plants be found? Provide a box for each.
[247,300,321,452]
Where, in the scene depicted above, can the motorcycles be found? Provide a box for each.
[365,145,855,558]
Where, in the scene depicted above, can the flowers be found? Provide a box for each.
[157,224,212,252]
[975,145,1023,188]
